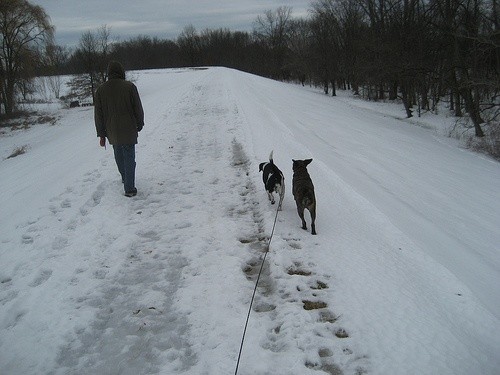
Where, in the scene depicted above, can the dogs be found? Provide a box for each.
[291,158,319,237]
[259,148,287,212]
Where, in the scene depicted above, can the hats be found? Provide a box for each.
[107,61,124,75]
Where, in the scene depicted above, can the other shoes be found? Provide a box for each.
[125,187,138,197]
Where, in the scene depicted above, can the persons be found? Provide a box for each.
[95,61,144,197]
